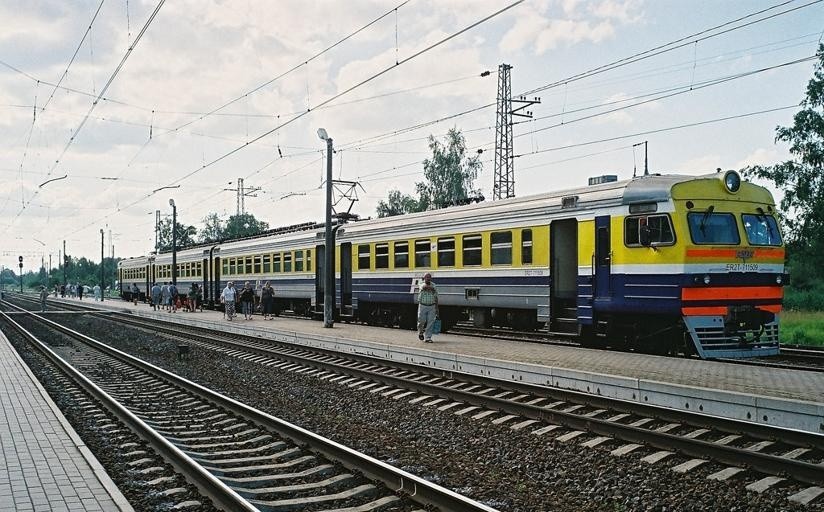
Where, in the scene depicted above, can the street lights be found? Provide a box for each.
[167,197,177,309]
[316,128,339,330]
[100,228,106,303]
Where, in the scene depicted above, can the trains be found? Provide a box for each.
[117,169,787,364]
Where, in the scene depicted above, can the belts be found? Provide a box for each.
[420,302,435,306]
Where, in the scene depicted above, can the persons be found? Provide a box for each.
[37,280,275,321]
[415,272,439,344]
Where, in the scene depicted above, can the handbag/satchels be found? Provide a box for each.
[433,316,441,334]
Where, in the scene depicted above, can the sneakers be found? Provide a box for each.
[227,315,237,321]
[426,340,433,343]
[245,317,252,320]
[153,306,177,313]
[263,317,274,320]
[419,335,424,340]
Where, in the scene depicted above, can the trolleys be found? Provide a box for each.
[182,293,203,312]
